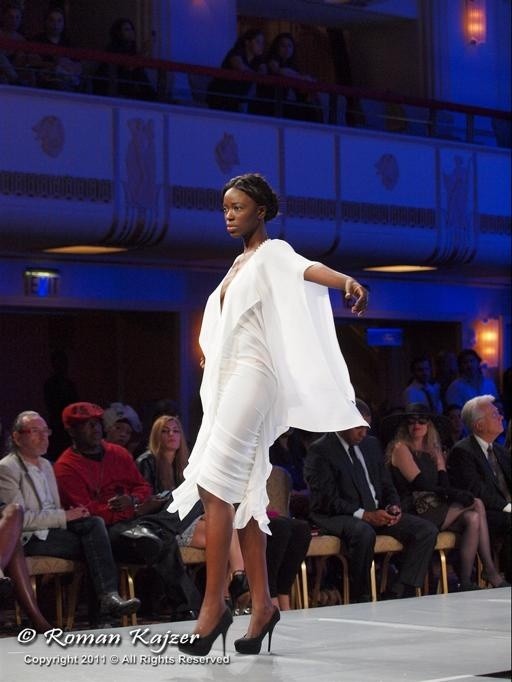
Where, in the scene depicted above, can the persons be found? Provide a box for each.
[175,172,370,657]
[1,5,28,82]
[91,16,157,101]
[28,7,80,83]
[206,30,265,110]
[248,31,323,120]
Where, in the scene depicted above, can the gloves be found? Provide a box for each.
[412,470,473,508]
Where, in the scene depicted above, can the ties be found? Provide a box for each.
[348,446,376,511]
[487,445,512,502]
[421,385,434,410]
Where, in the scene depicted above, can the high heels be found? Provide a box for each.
[480,569,510,587]
[234,605,280,653]
[179,607,233,657]
[229,574,250,609]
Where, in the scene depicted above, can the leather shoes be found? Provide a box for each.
[96,591,142,621]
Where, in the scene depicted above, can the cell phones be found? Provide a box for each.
[387,512,400,515]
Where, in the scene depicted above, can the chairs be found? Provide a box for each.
[12,530,460,633]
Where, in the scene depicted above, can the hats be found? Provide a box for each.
[62,403,105,428]
[382,403,453,429]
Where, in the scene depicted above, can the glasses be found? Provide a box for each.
[407,416,429,425]
[25,428,52,436]
